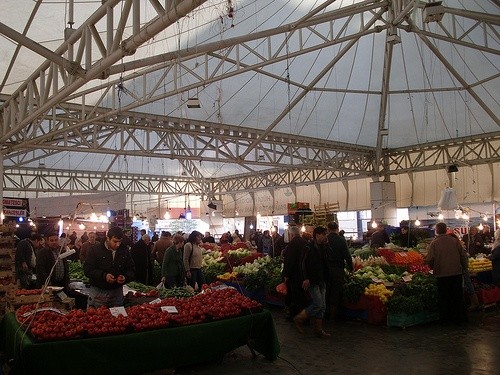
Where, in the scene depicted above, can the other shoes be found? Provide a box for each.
[292,315,305,334]
[314,329,332,339]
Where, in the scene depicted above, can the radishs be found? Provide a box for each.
[352,255,388,265]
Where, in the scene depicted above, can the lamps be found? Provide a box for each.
[448,166,458,172]
[179,214,186,222]
[258,149,265,160]
[187,87,201,108]
[39,157,46,169]
[58,244,76,260]
[208,203,217,210]
[106,210,117,218]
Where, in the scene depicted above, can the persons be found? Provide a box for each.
[425,223,477,333]
[392,226,419,248]
[9,218,57,240]
[131,234,153,283]
[139,229,215,244]
[84,227,131,303]
[183,231,205,293]
[371,224,391,248]
[37,231,70,290]
[489,230,500,290]
[279,225,309,320]
[321,222,353,320]
[292,227,329,336]
[160,235,185,288]
[154,232,174,268]
[220,225,287,256]
[15,232,43,288]
[463,224,498,255]
[58,230,101,267]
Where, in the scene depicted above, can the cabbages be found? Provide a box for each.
[350,264,398,281]
[203,248,271,279]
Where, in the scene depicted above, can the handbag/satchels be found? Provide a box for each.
[183,257,191,273]
[276,283,288,296]
[156,282,165,289]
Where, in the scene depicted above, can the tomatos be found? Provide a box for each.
[15,288,51,324]
[187,281,262,320]
[29,289,207,339]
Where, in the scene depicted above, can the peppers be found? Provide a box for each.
[127,281,199,299]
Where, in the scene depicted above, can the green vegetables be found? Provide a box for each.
[233,256,284,284]
[343,275,374,294]
[67,261,89,281]
[380,263,436,312]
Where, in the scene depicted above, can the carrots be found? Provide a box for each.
[393,251,423,263]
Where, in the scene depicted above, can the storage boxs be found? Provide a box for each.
[0,221,16,292]
[476,286,500,304]
[388,310,439,331]
[5,287,53,312]
[303,200,340,226]
